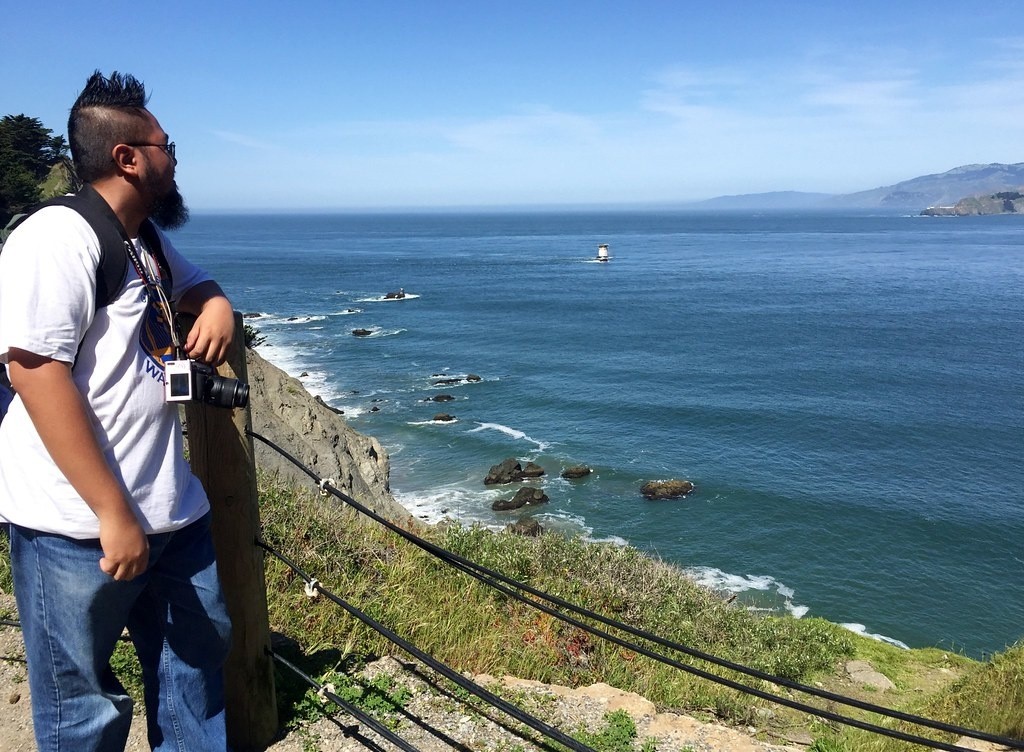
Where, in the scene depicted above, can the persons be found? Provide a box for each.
[1,70,235,752]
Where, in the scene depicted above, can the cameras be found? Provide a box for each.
[164,357,195,403]
[186,358,251,411]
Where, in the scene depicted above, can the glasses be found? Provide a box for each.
[110,142,176,161]
